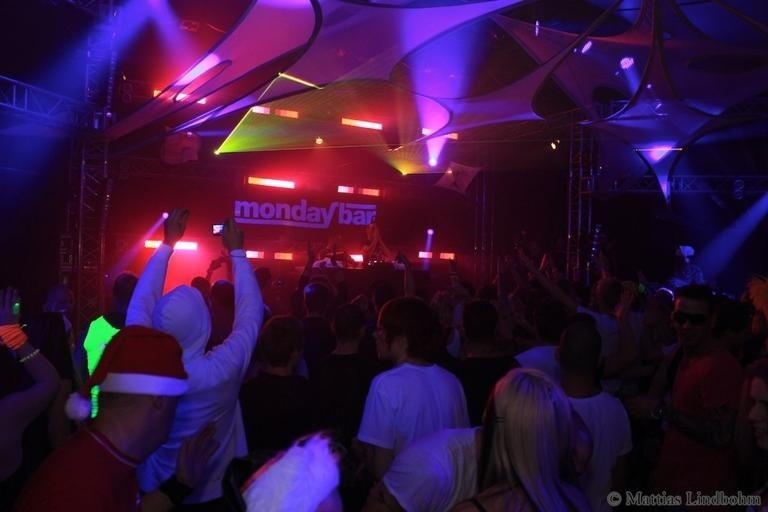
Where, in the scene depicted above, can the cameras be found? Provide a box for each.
[212,223,224,236]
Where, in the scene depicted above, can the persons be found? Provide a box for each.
[1,206,768,509]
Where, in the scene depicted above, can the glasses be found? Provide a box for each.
[671,310,708,326]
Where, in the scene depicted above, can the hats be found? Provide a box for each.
[80,325,191,401]
